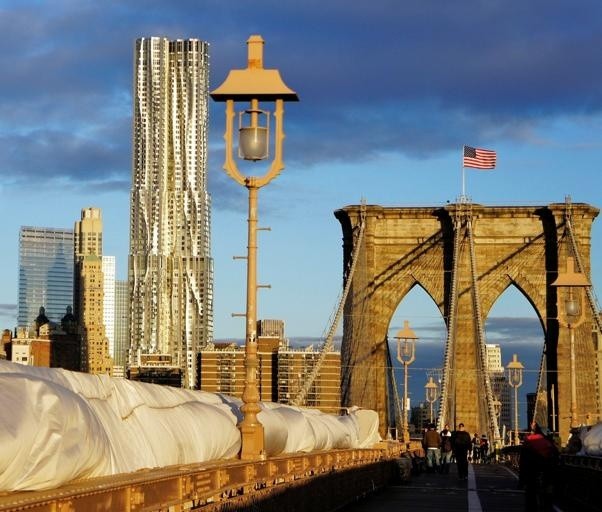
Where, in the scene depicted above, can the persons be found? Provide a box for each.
[450,422,473,484]
[420,423,444,473]
[437,424,490,470]
[517,420,568,512]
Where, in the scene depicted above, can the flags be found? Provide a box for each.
[464,145,497,171]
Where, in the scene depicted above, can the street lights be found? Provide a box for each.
[423,375,439,423]
[207,32,300,459]
[505,354,525,446]
[547,255,593,428]
[392,316,421,444]
[492,393,503,420]
